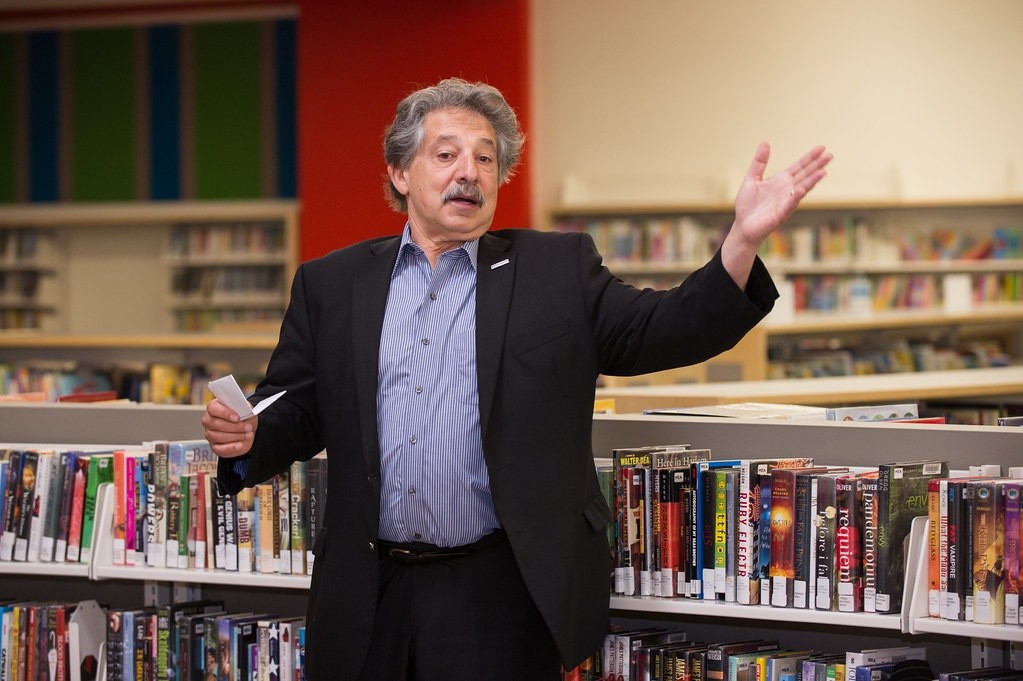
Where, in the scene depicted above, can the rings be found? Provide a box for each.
[791,190,794,197]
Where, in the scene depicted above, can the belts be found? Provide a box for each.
[377,542,468,566]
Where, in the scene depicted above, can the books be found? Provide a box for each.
[169,221,287,334]
[633,401,1023,435]
[0,599,307,681]
[0,440,329,575]
[587,446,1023,626]
[564,617,1023,681]
[769,338,1008,377]
[0,224,64,329]
[556,212,1023,305]
[0,357,257,408]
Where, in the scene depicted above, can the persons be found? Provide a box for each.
[202,77,834,681]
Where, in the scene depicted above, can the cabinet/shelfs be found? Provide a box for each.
[0,200,1022,681]
